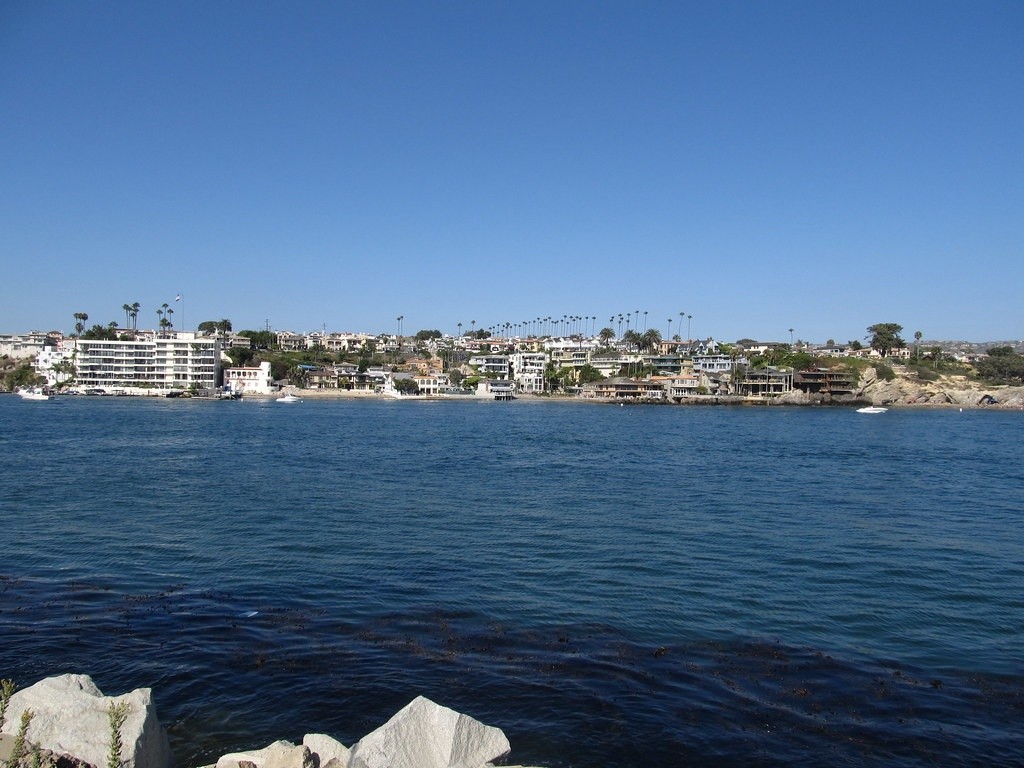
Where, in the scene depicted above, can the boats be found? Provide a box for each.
[275,396,301,403]
[856,406,889,414]
[18,387,55,401]
